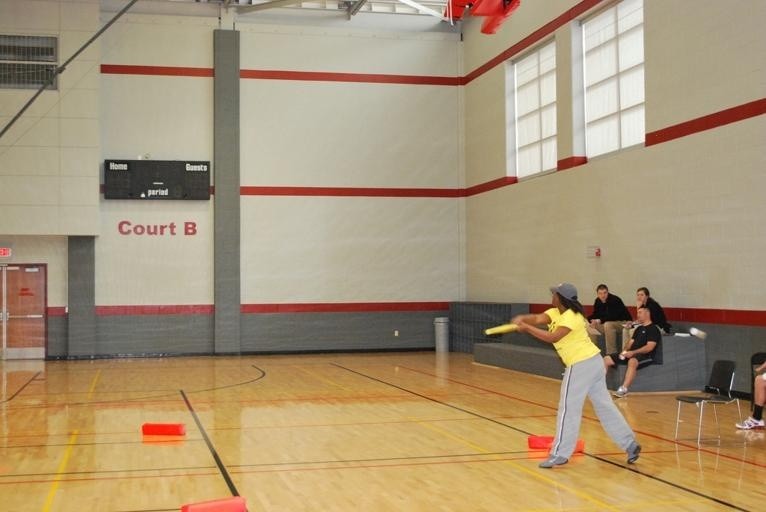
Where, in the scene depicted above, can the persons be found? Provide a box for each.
[511,282,642,468]
[586,284,672,397]
[736,360,766,430]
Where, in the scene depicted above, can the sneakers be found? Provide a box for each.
[612,386,628,397]
[735,416,765,430]
[627,441,641,464]
[539,454,567,468]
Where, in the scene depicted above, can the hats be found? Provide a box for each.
[549,281,577,302]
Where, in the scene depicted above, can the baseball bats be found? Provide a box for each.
[486,323,519,336]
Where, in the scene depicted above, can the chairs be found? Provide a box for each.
[672,353,766,446]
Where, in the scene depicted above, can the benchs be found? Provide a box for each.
[470,332,705,395]
